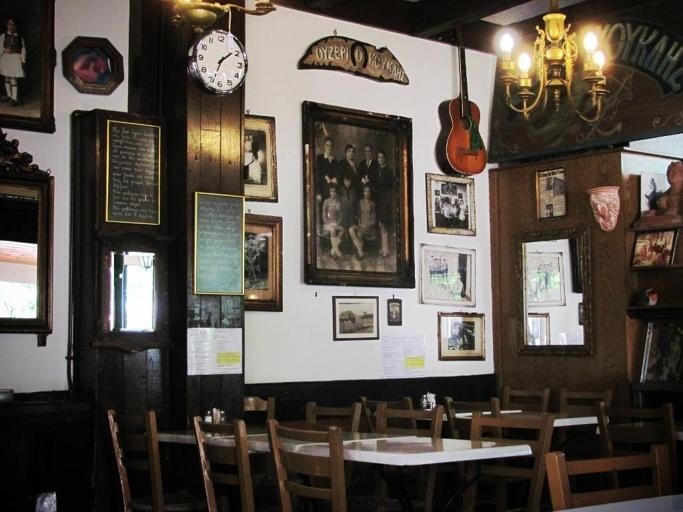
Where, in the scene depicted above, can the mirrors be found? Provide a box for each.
[513,224,596,359]
[95,231,171,355]
[2,130,54,346]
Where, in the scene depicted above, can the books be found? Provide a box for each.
[639,319,682,383]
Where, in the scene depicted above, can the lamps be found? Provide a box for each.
[483,0,619,127]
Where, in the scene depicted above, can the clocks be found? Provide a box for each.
[187,8,250,96]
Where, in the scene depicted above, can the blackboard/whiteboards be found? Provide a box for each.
[105,120,161,226]
[193,192,245,296]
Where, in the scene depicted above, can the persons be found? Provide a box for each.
[93,56,113,86]
[244,234,261,289]
[320,183,346,260]
[432,182,466,228]
[428,255,448,283]
[634,231,666,265]
[337,144,360,196]
[389,304,399,322]
[0,15,28,106]
[337,174,359,240]
[655,161,682,216]
[242,132,262,185]
[658,328,682,382]
[348,185,376,260]
[368,150,394,259]
[316,136,338,246]
[358,143,378,185]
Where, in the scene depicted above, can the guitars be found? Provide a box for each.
[436,26,487,175]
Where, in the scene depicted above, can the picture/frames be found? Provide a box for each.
[567,234,587,293]
[524,310,550,347]
[1,1,57,133]
[523,250,567,307]
[533,165,568,224]
[576,302,586,327]
[628,225,680,269]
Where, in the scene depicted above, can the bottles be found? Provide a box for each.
[203,410,212,437]
[420,394,427,409]
[220,410,225,424]
